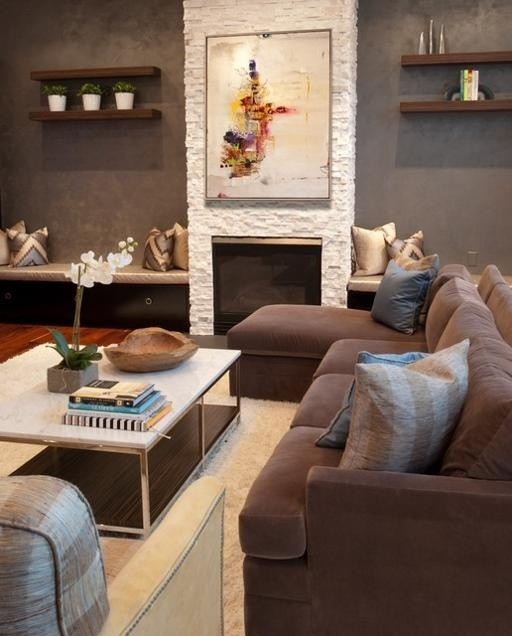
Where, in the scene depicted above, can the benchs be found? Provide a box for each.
[0,263,190,332]
[346,268,471,310]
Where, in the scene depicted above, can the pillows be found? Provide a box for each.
[144,226,174,271]
[7,225,50,266]
[401,253,440,282]
[173,220,188,270]
[333,333,472,476]
[381,227,430,276]
[0,216,31,265]
[347,220,402,277]
[365,254,428,339]
[311,344,429,452]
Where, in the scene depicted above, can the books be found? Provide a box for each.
[61,378,174,440]
[459,68,478,101]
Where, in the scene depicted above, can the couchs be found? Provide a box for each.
[240,266,512,634]
[225,258,484,404]
[0,465,227,636]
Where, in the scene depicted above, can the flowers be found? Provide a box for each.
[46,233,138,361]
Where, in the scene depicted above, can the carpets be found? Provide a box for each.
[0,336,307,636]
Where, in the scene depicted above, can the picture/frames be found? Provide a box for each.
[204,26,334,202]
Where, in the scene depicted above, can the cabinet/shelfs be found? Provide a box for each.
[399,50,511,113]
[27,65,162,122]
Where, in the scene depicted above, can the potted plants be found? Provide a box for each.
[112,79,139,113]
[77,81,106,111]
[44,81,70,113]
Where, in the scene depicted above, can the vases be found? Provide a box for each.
[45,364,101,394]
